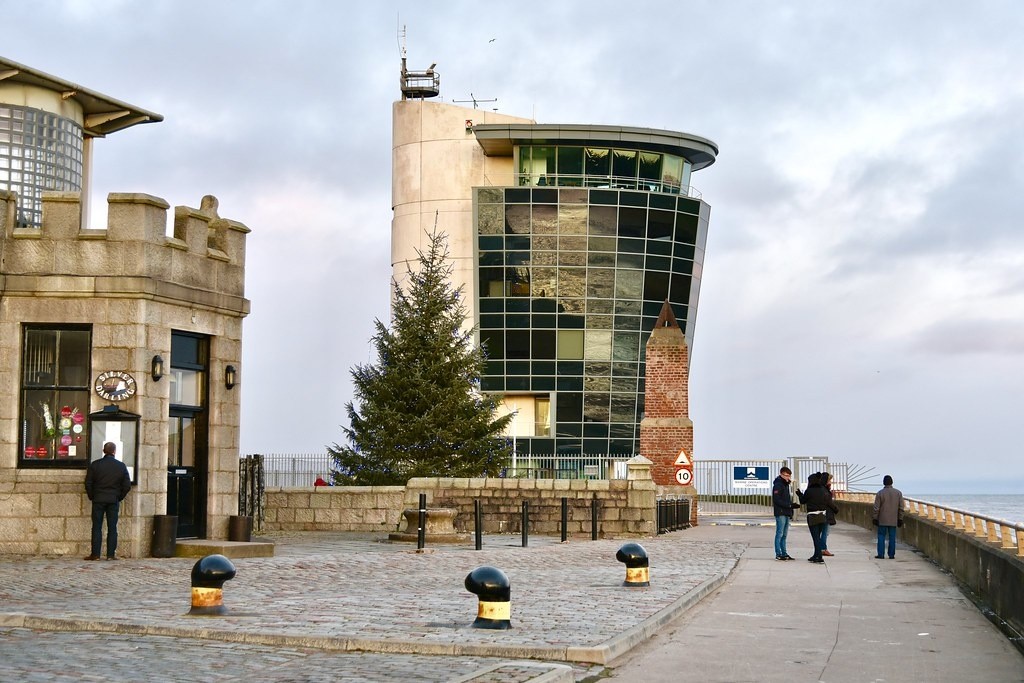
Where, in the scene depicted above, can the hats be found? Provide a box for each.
[884,475,894,485]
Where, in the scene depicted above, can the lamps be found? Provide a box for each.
[152,356,164,381]
[226,364,236,389]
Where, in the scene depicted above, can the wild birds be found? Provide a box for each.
[488,39,497,43]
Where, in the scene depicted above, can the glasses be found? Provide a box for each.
[783,474,792,478]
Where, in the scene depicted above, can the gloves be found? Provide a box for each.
[834,507,839,515]
[791,503,801,510]
[795,488,803,497]
[874,519,879,526]
[897,520,903,528]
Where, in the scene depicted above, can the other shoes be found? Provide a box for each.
[777,556,788,561]
[785,554,795,560]
[889,556,896,559]
[107,556,119,561]
[822,551,835,556]
[84,555,100,561]
[807,557,817,560]
[875,555,884,559]
[812,559,824,564]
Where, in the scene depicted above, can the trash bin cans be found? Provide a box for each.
[228,515,253,542]
[151,515,178,558]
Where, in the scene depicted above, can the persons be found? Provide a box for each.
[772,466,801,561]
[795,472,839,563]
[83,442,131,561]
[314,474,327,486]
[872,475,905,560]
